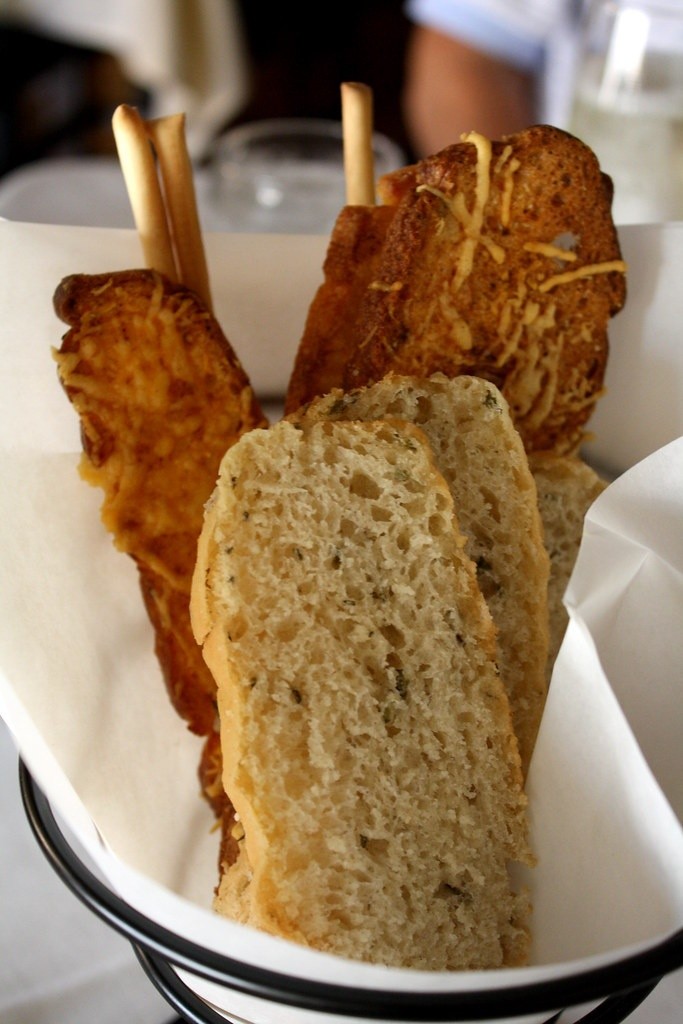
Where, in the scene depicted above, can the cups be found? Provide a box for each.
[558,0,682,223]
[202,118,402,243]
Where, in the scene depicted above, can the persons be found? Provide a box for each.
[403,0,683,158]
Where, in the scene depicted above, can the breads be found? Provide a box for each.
[191,373,626,971]
